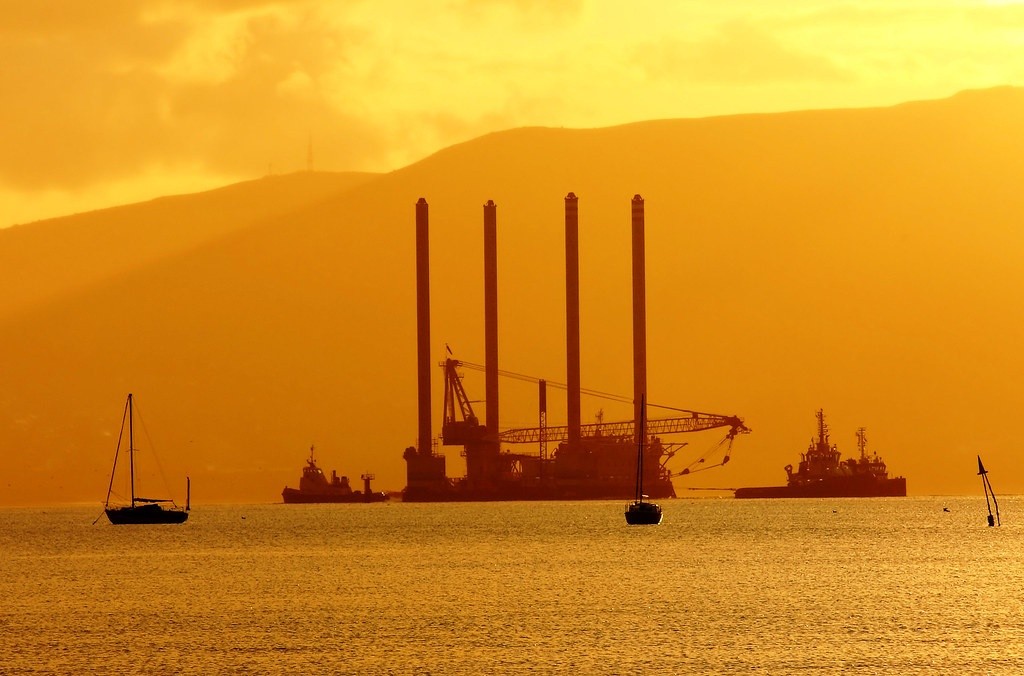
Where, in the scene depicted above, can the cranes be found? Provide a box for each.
[438,343,742,478]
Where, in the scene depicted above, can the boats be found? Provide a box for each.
[281,444,390,505]
[734,443,908,500]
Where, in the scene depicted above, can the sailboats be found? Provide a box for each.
[623,393,664,524]
[104,392,190,525]
